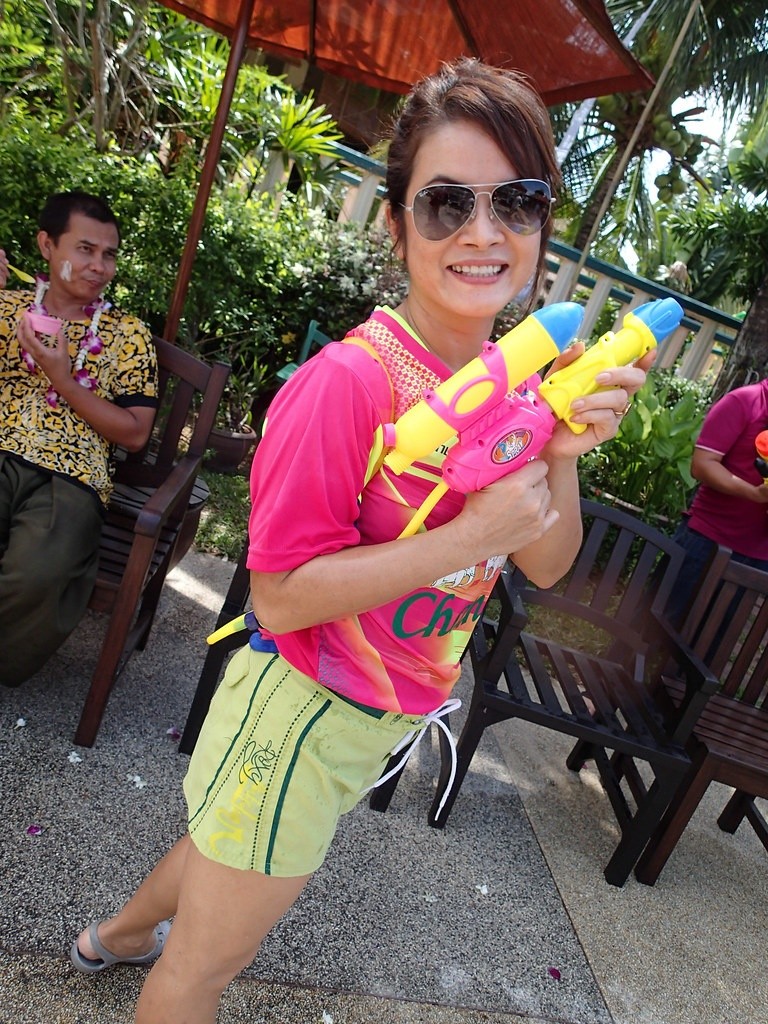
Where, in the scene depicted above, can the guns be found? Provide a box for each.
[382,296,685,495]
[754,429,768,485]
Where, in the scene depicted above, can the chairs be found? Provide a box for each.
[429,495,719,886]
[75,333,230,747]
[634,546,768,886]
[179,531,420,810]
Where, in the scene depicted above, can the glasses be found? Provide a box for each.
[401,178,558,241]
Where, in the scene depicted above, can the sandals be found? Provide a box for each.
[70,917,172,973]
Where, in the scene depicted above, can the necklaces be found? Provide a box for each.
[21,275,112,407]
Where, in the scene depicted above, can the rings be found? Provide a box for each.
[614,402,632,416]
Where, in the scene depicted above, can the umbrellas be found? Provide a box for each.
[161,1,656,340]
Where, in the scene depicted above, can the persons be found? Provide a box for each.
[0,188,160,689]
[68,54,657,1024]
[605,377,768,680]
[428,187,443,222]
[506,192,522,216]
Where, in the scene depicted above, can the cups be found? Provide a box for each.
[24,310,62,348]
